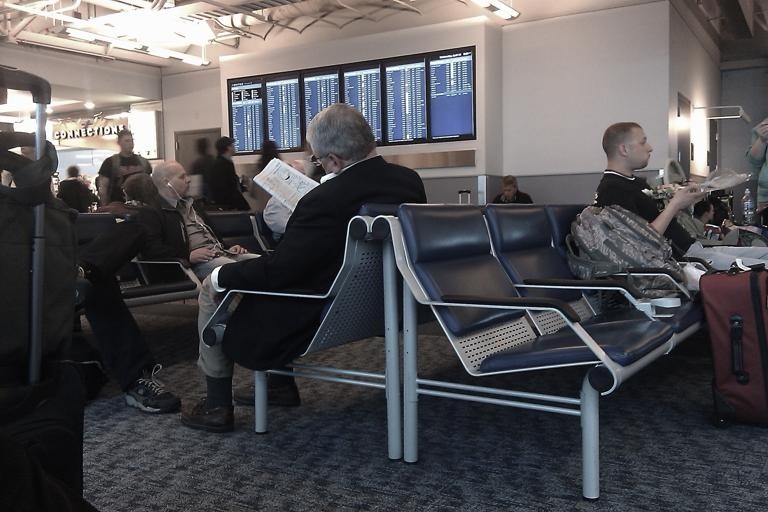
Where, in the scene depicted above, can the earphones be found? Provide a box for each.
[168,182,172,187]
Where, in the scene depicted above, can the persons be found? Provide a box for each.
[490,177,535,204]
[176,105,426,433]
[592,116,768,271]
[1,103,329,412]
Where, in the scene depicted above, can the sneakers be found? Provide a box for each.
[124,377,182,414]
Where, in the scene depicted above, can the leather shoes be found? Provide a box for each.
[235,386,301,407]
[179,399,234,433]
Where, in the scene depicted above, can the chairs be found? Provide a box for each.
[64,201,767,503]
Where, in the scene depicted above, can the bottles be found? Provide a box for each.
[741,188,754,223]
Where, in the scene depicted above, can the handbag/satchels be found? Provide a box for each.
[566,207,687,297]
[1,185,75,388]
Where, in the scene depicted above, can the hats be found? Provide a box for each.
[216,137,235,149]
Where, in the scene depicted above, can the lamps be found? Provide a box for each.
[691,105,750,123]
[455,0,521,23]
[58,28,212,69]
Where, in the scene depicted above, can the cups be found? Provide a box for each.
[91,203,99,212]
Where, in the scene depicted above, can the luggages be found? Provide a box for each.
[0,65,83,512]
[700,261,768,427]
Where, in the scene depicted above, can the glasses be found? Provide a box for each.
[311,155,326,167]
